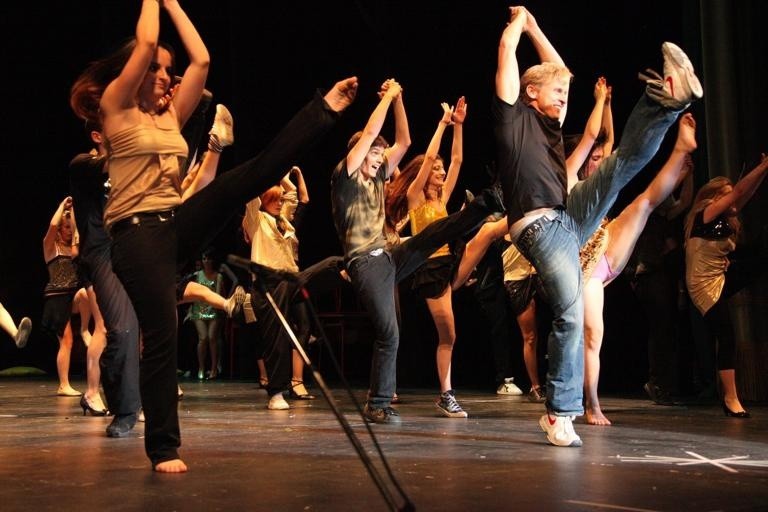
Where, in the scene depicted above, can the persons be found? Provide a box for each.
[248,165,317,400]
[80,258,112,417]
[475,177,524,396]
[331,77,508,425]
[366,166,411,401]
[680,148,768,417]
[626,159,694,405]
[489,1,711,446]
[179,258,238,379]
[187,249,225,380]
[64,75,238,435]
[93,0,364,474]
[499,233,546,403]
[240,177,347,412]
[42,196,83,396]
[0,301,32,348]
[386,94,509,417]
[563,76,697,425]
[174,279,246,398]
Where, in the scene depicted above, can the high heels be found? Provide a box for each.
[259,376,269,388]
[290,379,315,400]
[724,398,752,418]
[80,393,113,416]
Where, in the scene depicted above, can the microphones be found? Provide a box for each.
[206,243,265,275]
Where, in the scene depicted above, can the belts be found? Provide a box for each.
[110,209,175,234]
[515,205,562,250]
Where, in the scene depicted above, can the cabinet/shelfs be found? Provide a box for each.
[228,280,368,381]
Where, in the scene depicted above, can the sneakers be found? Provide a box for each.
[208,103,235,149]
[106,413,138,437]
[198,371,204,380]
[484,173,506,213]
[228,286,246,317]
[208,375,217,380]
[360,382,587,447]
[639,41,703,106]
[16,317,32,348]
[178,385,184,400]
